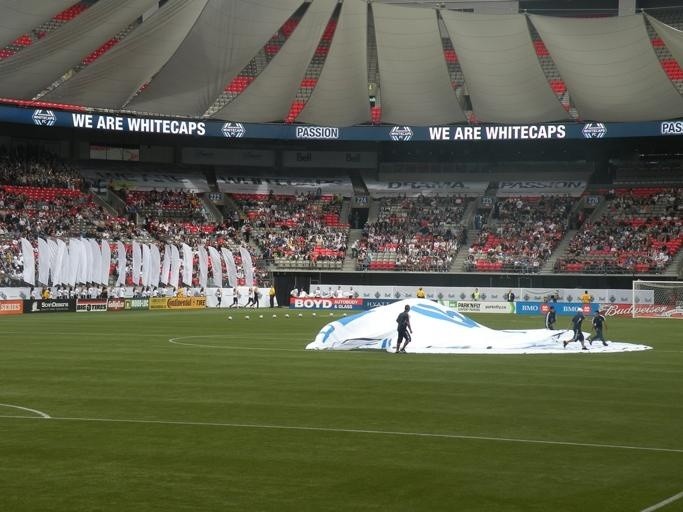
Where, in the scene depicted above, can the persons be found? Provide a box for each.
[215,285,276,308]
[30,284,205,299]
[551,292,558,302]
[507,290,515,302]
[472,288,480,301]
[563,306,609,349]
[397,305,413,354]
[579,288,591,302]
[290,287,356,298]
[416,287,424,298]
[546,305,556,329]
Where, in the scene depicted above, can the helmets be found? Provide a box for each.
[577,308,583,313]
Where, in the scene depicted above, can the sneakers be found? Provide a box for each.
[582,346,589,350]
[602,342,608,346]
[589,339,592,346]
[563,340,567,350]
[395,348,407,354]
